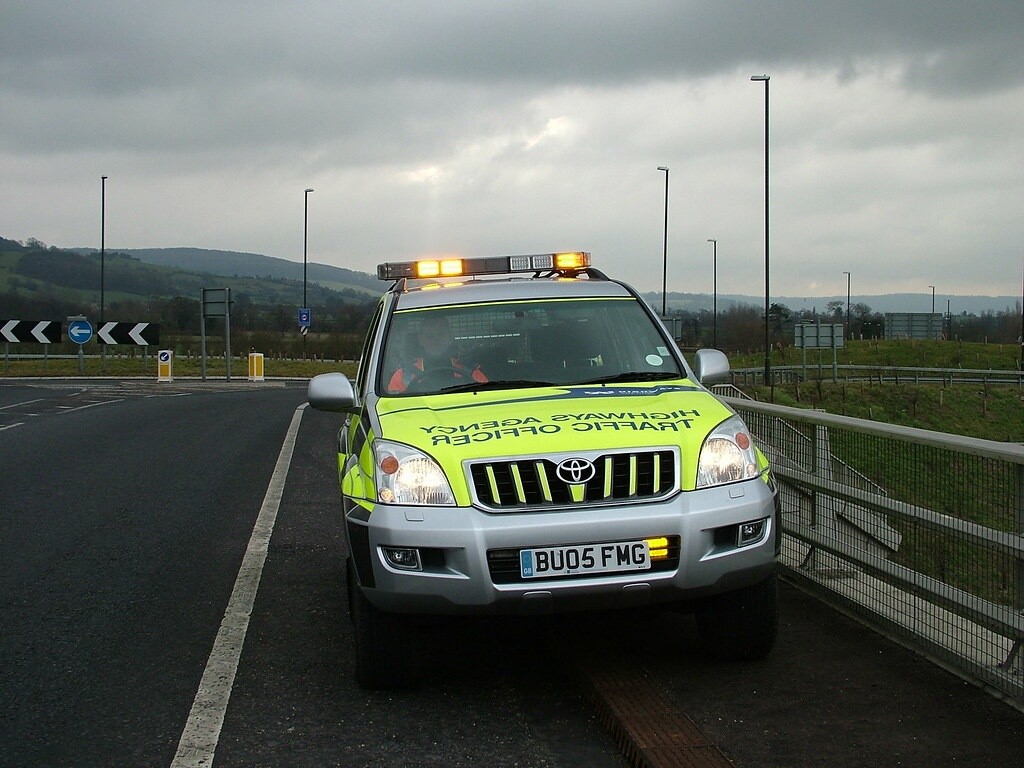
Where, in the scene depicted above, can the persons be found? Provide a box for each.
[387,317,488,394]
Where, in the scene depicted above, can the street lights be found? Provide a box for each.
[99,176,110,360]
[750,74,771,386]
[842,271,850,341]
[706,237,717,348]
[302,186,315,361]
[928,285,935,313]
[656,166,669,318]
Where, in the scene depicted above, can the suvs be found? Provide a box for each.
[307,251,784,643]
